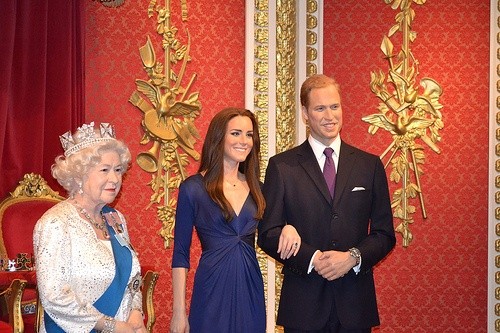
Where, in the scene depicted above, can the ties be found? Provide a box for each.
[322,147,338,201]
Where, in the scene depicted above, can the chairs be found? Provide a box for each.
[0,172,158,333]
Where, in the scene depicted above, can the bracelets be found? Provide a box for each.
[349,248,360,263]
[102,317,116,333]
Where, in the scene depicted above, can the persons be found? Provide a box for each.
[260,74,397,333]
[169,107,302,333]
[33,122,151,333]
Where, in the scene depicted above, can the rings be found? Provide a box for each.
[294,242,299,249]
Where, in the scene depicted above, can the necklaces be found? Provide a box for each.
[75,200,111,239]
[228,179,240,188]
